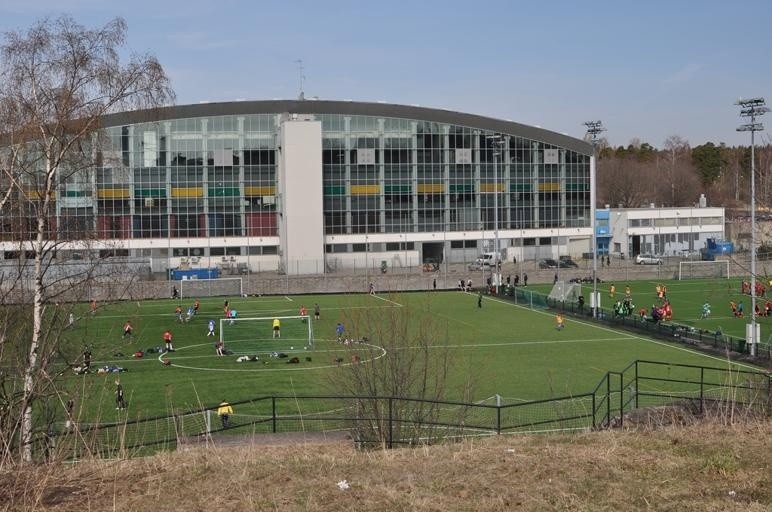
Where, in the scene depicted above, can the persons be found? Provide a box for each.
[602,255,604,267]
[699,280,772,320]
[513,256,516,266]
[66,286,344,428]
[369,282,376,295]
[457,272,673,331]
[607,255,611,266]
[498,259,504,271]
[433,278,436,289]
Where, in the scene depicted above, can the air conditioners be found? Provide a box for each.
[180,257,188,263]
[191,257,198,262]
[221,256,228,261]
[230,256,236,260]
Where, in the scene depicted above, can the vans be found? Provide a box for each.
[475,251,502,266]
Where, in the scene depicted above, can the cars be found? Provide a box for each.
[538,258,578,269]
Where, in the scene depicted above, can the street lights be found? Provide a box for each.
[580,120,610,321]
[732,95,771,354]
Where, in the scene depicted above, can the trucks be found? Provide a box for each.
[422,258,435,272]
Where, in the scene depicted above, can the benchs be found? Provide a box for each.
[680,336,702,345]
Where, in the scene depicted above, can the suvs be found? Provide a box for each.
[636,254,664,264]
